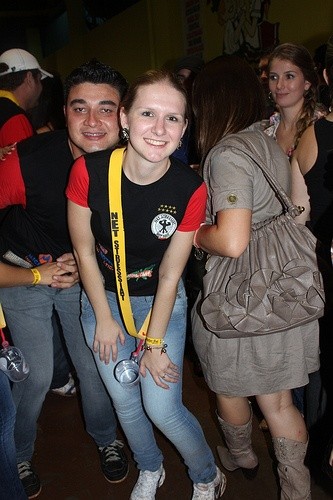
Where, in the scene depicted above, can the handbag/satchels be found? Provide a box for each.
[201,135,325,340]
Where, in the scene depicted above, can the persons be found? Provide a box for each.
[263,43,333,489]
[183,58,325,500]
[66,70,226,500]
[0,48,129,500]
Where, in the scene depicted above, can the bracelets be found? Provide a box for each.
[31,268,40,285]
[194,223,209,248]
[145,338,168,353]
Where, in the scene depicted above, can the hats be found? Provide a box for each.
[0,48,54,80]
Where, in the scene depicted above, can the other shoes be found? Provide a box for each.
[51,374,76,397]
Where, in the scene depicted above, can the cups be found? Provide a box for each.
[113,353,141,389]
[0,346,31,383]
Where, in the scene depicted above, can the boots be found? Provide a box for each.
[217,400,258,481]
[272,433,310,500]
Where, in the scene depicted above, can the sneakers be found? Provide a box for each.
[18,460,42,499]
[98,439,128,482]
[190,465,227,500]
[129,463,165,500]
